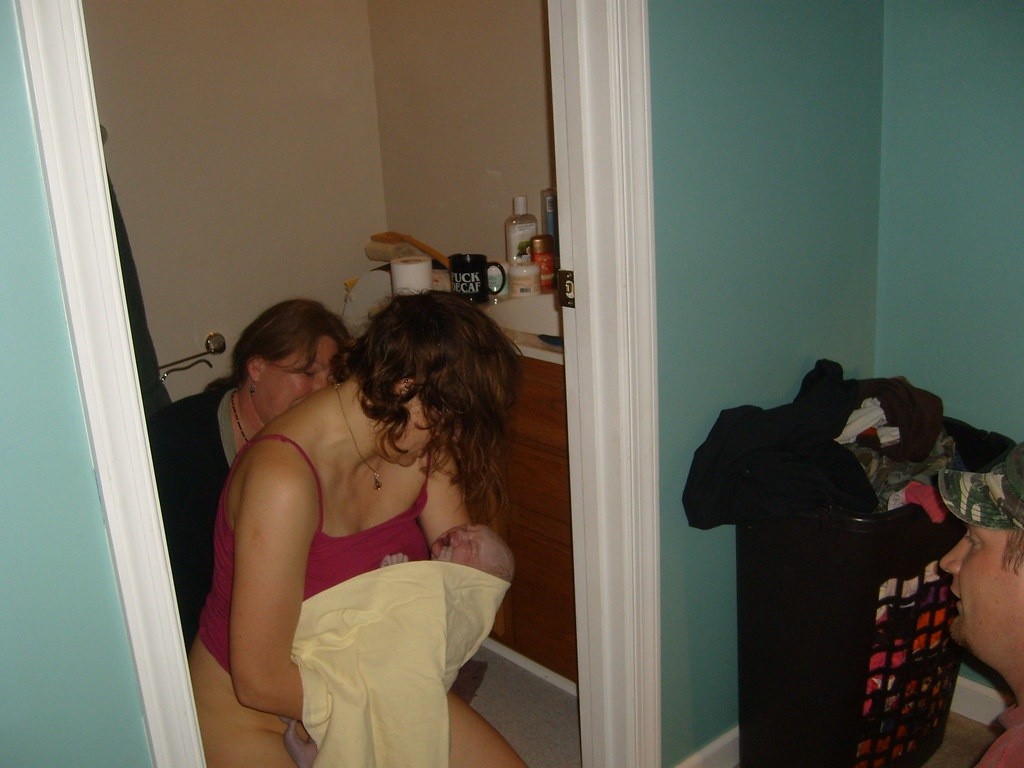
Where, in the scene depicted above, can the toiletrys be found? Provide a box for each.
[505,195,538,262]
[542,168,559,257]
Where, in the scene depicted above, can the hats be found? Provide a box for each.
[938,439,1024,531]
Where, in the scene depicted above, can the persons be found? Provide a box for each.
[190,289,526,767]
[937,441,1024,768]
[149,299,350,625]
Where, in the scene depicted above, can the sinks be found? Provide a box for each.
[482,292,563,337]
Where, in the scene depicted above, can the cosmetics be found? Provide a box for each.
[488,195,558,296]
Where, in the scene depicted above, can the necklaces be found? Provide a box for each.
[332,382,386,491]
[230,391,249,443]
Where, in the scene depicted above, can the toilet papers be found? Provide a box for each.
[391,256,432,295]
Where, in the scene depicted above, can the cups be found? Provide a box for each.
[446,254,505,306]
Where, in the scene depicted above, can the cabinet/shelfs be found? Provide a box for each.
[478,332,578,699]
